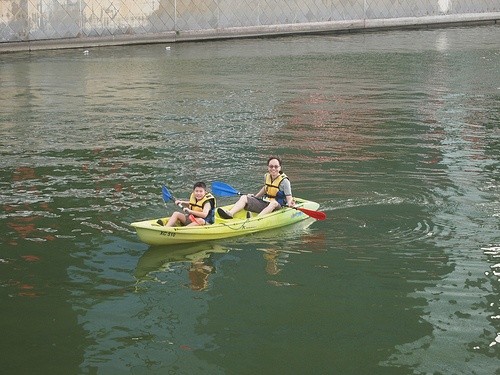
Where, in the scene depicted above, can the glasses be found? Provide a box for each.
[267,165,280,168]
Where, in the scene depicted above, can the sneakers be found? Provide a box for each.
[217,207,233,218]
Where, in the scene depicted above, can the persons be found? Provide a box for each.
[264,240,291,275]
[188,255,215,289]
[217,157,295,219]
[156,182,216,227]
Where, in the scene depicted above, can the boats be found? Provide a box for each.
[129,196,320,246]
[134,216,315,281]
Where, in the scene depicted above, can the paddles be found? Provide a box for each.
[162,184,201,225]
[211,181,326,220]
